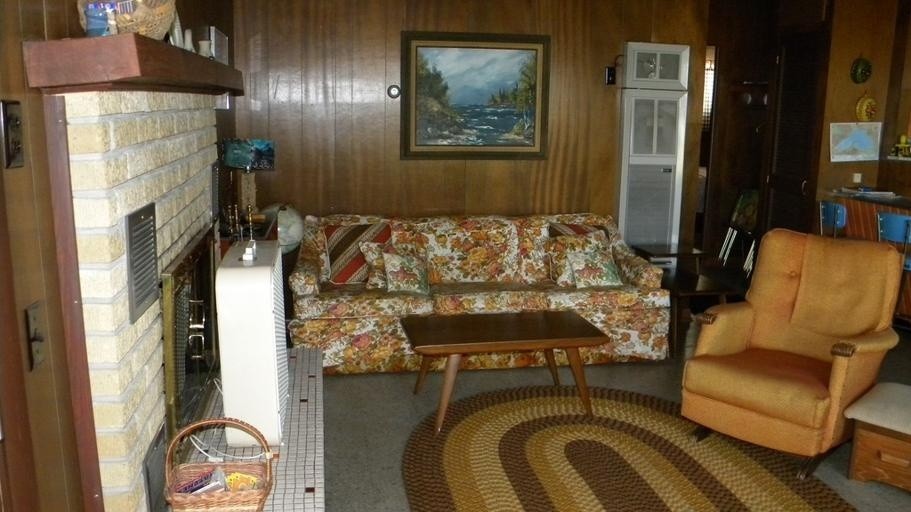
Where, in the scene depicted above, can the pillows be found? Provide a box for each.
[516,216,626,283]
[357,240,438,290]
[320,222,393,288]
[388,215,529,286]
[382,243,431,294]
[543,231,612,287]
[563,245,624,288]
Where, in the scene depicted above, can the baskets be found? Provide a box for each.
[78,1,175,41]
[164,417,272,512]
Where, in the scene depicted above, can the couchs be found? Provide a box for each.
[286,209,675,376]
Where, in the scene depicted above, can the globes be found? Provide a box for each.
[261,203,304,255]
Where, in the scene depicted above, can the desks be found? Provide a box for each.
[399,309,612,433]
[628,240,740,348]
[831,186,911,323]
[846,378,911,495]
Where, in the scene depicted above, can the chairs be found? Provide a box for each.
[681,226,905,480]
[820,200,849,237]
[875,208,910,335]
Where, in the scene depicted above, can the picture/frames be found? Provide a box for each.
[397,26,553,163]
[207,23,231,112]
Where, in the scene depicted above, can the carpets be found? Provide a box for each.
[401,383,860,512]
